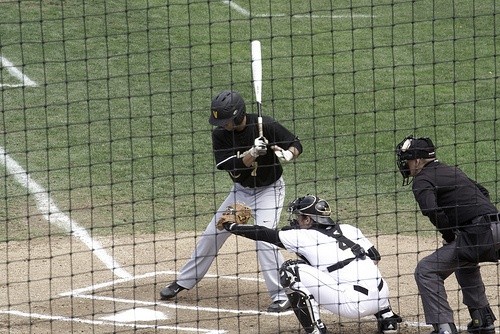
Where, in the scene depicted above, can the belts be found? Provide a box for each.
[483,214,500,222]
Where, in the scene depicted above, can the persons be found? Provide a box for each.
[160,89,303,313]
[220,194,403,334]
[395,134,500,334]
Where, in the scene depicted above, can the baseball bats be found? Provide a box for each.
[250,39,266,138]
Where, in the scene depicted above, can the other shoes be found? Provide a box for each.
[467,319,495,333]
[429,322,458,334]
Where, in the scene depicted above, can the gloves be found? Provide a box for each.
[270,144,295,162]
[250,137,267,158]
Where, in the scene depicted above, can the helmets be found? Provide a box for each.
[394,134,436,185]
[208,89,246,125]
[286,194,336,227]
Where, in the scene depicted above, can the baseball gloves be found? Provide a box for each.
[215,202,254,229]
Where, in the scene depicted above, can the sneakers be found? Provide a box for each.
[380,313,402,334]
[267,299,293,312]
[308,320,327,334]
[160,281,186,297]
